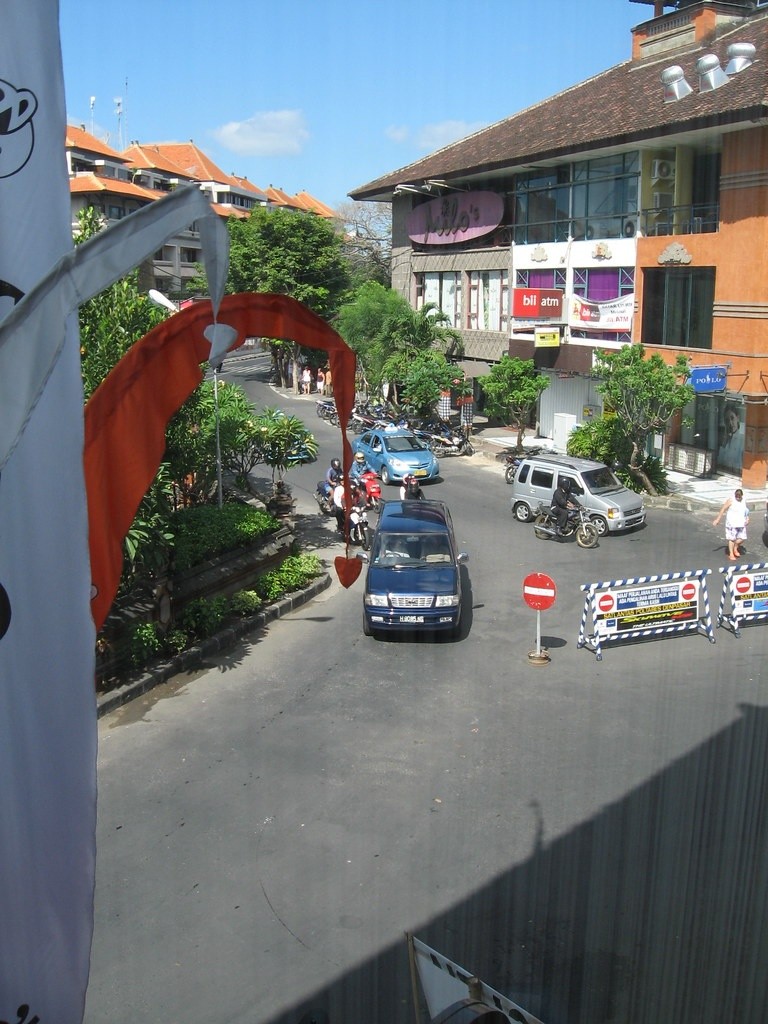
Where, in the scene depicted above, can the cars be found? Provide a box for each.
[511,455,646,537]
[351,423,439,485]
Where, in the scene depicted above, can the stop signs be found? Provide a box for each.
[599,595,614,612]
[523,573,557,610]
[682,584,695,600]
[736,577,751,593]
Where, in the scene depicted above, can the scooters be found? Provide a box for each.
[316,397,475,458]
[313,470,385,551]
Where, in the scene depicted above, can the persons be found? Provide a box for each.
[334,474,370,540]
[348,452,378,486]
[303,365,311,395]
[716,405,745,471]
[400,473,425,501]
[271,355,276,371]
[550,477,581,535]
[325,368,333,397]
[317,368,325,395]
[713,489,749,561]
[324,458,343,509]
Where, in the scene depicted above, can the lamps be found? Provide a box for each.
[393,179,468,198]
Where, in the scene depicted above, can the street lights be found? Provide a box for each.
[148,289,222,509]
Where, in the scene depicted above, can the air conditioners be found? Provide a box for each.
[587,223,609,239]
[651,159,675,180]
[623,218,639,239]
[653,192,673,213]
[667,445,712,472]
[692,218,703,233]
[681,220,690,232]
[654,222,674,236]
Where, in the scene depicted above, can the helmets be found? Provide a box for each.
[558,477,570,489]
[409,479,419,493]
[349,481,356,493]
[403,473,412,484]
[336,474,344,483]
[330,458,340,470]
[355,452,365,462]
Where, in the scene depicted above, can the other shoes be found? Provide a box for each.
[337,525,343,531]
[323,503,332,512]
[734,550,740,556]
[729,555,737,561]
[560,527,568,532]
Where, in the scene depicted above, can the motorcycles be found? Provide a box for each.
[505,449,540,484]
[533,500,598,548]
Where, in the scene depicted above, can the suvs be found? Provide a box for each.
[356,499,469,637]
